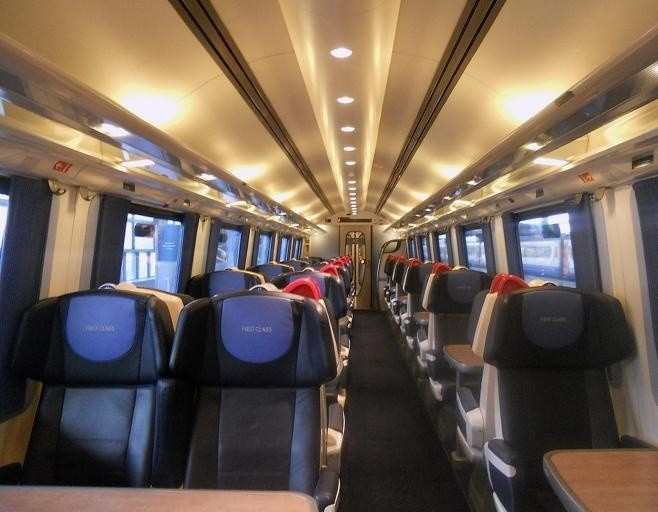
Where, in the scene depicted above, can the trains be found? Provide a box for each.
[423,236,578,283]
